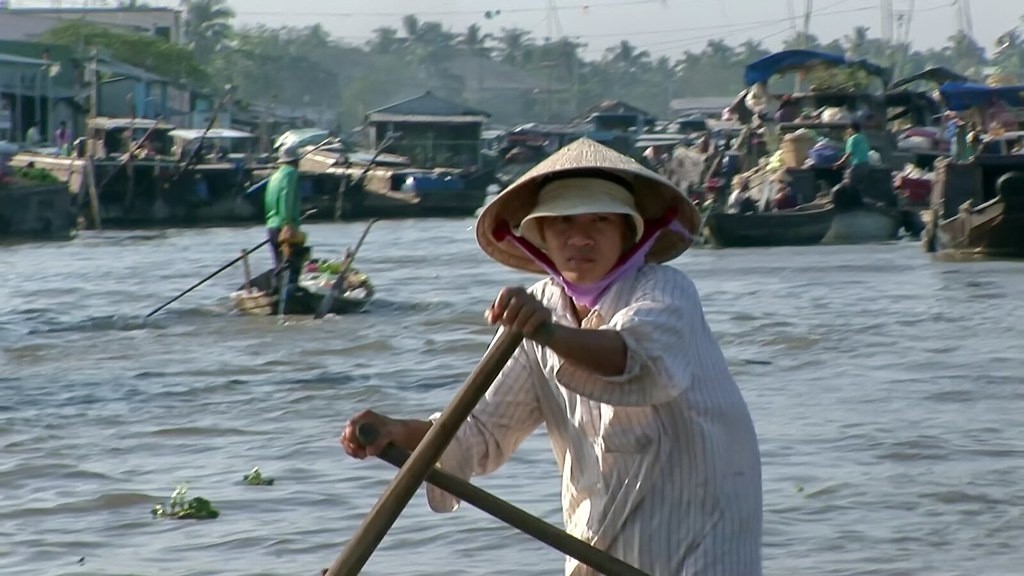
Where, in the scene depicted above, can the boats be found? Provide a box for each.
[235,258,375,315]
[8,48,1024,262]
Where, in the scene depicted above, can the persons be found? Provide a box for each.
[24,121,42,148]
[53,120,74,147]
[262,143,303,316]
[830,120,870,202]
[340,134,764,576]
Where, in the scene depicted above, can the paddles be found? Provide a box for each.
[144,208,319,318]
[318,299,555,576]
[359,424,649,576]
[315,217,381,319]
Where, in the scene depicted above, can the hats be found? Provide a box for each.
[277,143,304,164]
[476,137,701,274]
[521,168,643,251]
